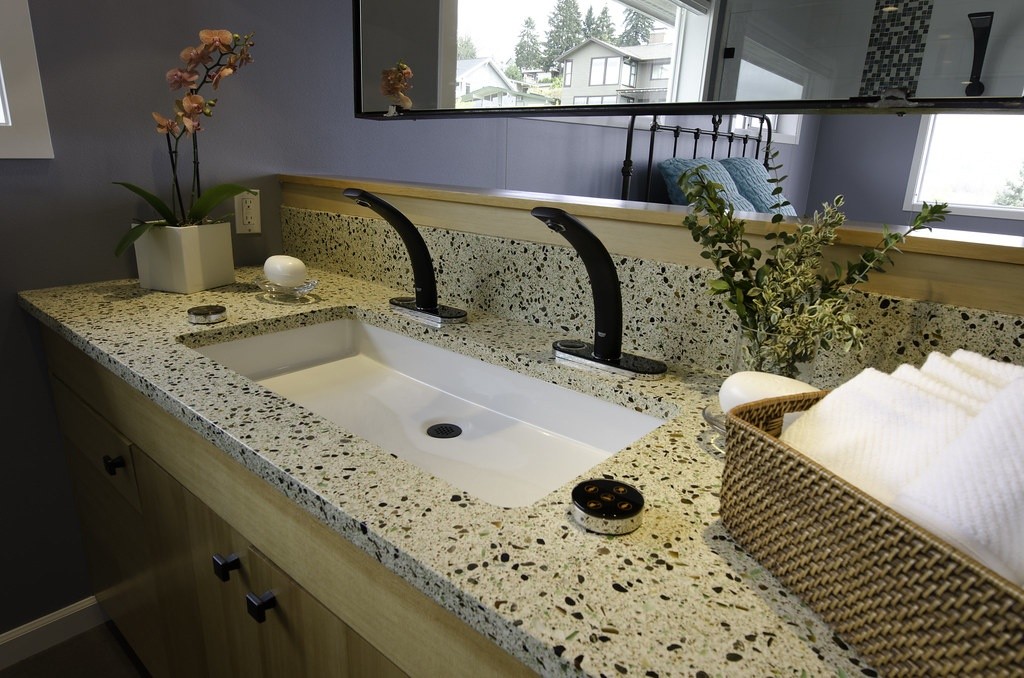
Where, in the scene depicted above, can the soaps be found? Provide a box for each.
[263,255,309,288]
[718,366,821,418]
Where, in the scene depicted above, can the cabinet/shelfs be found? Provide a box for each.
[34,315,532,678]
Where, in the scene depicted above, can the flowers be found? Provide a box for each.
[109,23,258,257]
[382,56,412,96]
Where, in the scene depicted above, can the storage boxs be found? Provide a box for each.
[720,393,1019,676]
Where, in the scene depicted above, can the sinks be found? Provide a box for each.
[172,305,688,515]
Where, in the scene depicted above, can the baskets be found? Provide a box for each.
[720,389,1024,678]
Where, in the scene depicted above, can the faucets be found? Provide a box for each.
[343,186,472,328]
[529,203,672,381]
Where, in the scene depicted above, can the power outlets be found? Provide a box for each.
[234,189,261,234]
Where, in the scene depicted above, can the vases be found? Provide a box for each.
[385,92,413,110]
[126,217,237,295]
[700,325,811,441]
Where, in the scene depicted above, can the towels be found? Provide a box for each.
[777,346,1024,599]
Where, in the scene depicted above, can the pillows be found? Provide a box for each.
[721,154,800,219]
[659,155,754,212]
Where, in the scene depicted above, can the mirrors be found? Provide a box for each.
[356,2,1023,121]
